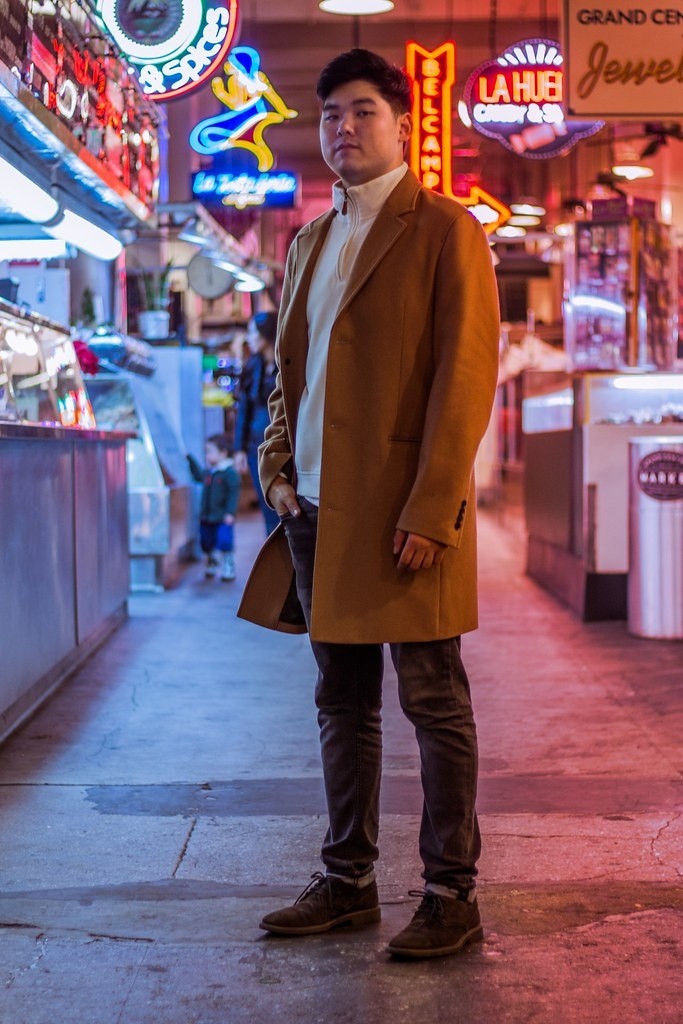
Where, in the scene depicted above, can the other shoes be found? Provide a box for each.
[220,553,237,580]
[204,552,217,579]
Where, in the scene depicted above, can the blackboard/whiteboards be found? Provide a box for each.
[559,0,683,120]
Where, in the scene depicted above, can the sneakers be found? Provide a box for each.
[258,869,382,936]
[384,881,485,959]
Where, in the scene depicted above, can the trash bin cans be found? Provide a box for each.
[625,436,683,642]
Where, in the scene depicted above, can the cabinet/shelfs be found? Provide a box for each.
[522,368,683,623]
[0,297,136,744]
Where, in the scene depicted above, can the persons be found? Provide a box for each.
[231,312,278,537]
[237,48,502,957]
[184,435,240,580]
